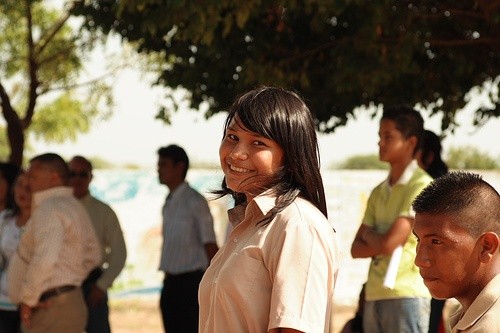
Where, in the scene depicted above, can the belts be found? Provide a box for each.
[39,284,75,300]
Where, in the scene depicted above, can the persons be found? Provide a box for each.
[65,157,127,333]
[157,144,219,333]
[0,160,24,215]
[198,85,340,333]
[0,170,32,333]
[411,171,500,333]
[6,153,103,333]
[350,105,437,333]
[412,130,450,178]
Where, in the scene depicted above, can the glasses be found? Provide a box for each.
[67,170,90,179]
